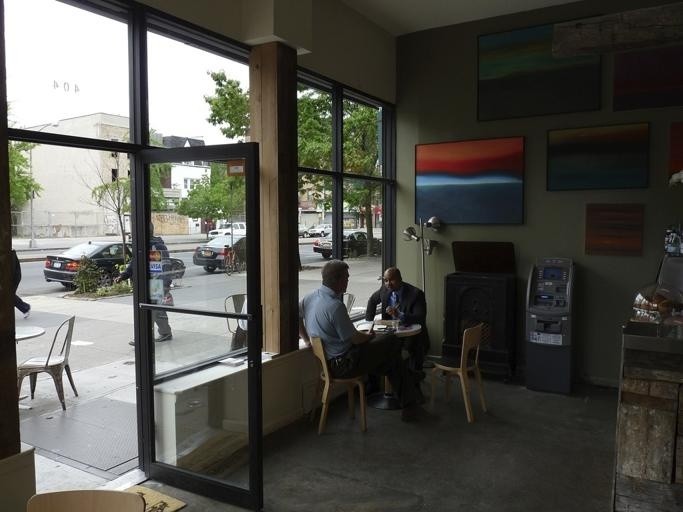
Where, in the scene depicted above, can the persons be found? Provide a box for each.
[114,222,172,346]
[299,260,429,422]
[12,250,30,319]
[366,267,430,386]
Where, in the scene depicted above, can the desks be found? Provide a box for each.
[15,326,45,341]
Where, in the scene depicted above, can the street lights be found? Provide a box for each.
[25,119,60,248]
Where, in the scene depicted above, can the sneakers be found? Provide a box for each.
[411,369,426,383]
[400,406,440,421]
[24,304,32,319]
[155,334,172,342]
[128,338,134,346]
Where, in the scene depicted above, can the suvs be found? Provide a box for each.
[207,221,246,237]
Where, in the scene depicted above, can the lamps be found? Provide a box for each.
[403,217,443,296]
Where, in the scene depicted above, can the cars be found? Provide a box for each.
[308,223,332,237]
[190,233,248,275]
[298,223,309,237]
[42,240,187,290]
[310,227,381,259]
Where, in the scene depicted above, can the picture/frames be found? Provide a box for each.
[546,121,650,190]
[415,136,525,225]
[669,122,683,180]
[584,203,644,256]
[612,44,683,112]
[477,14,602,122]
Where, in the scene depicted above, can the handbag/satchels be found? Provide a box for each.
[158,293,173,306]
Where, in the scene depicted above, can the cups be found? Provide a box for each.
[392,322,398,331]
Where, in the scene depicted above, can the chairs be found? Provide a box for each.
[310,337,368,436]
[429,323,488,423]
[16,315,78,410]
[224,293,249,351]
